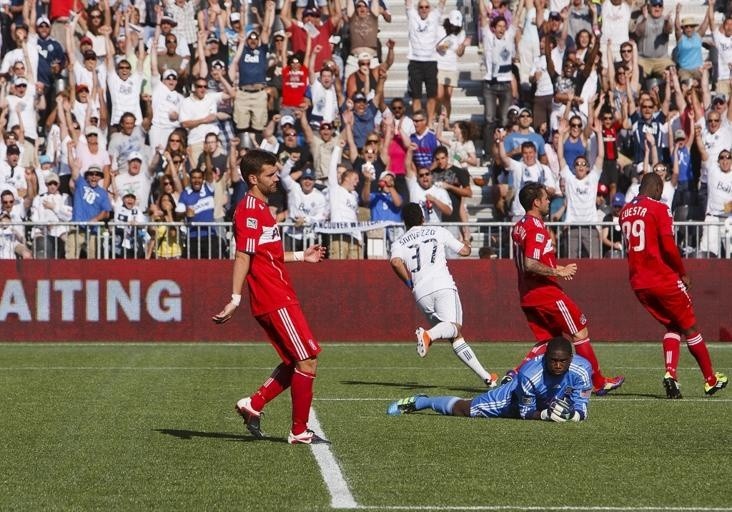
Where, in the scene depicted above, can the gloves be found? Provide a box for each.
[540,397,582,423]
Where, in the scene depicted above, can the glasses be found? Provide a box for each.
[282,133,296,137]
[419,5,429,10]
[506,49,730,173]
[2,199,14,203]
[89,172,98,177]
[47,182,57,185]
[160,137,184,186]
[319,106,431,181]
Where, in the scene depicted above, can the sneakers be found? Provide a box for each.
[415,326,432,357]
[483,372,498,387]
[287,427,330,445]
[704,372,727,394]
[387,393,429,415]
[500,369,519,382]
[235,395,268,440]
[663,372,681,400]
[594,374,625,395]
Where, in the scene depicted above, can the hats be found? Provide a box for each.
[612,191,625,207]
[648,1,662,6]
[280,115,294,126]
[12,0,382,103]
[680,18,700,28]
[598,181,607,194]
[301,167,314,181]
[84,126,97,136]
[548,12,560,20]
[83,166,103,178]
[449,10,462,29]
[129,151,141,162]
[44,172,60,186]
[6,145,19,155]
[120,187,134,200]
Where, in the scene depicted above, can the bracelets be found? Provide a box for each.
[230,293,242,308]
[571,412,581,421]
[405,280,412,287]
[540,409,548,420]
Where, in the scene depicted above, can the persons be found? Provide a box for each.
[618,172,728,399]
[389,202,497,389]
[387,337,593,421]
[213,149,330,445]
[500,183,625,396]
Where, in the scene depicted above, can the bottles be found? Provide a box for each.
[425,196,433,215]
